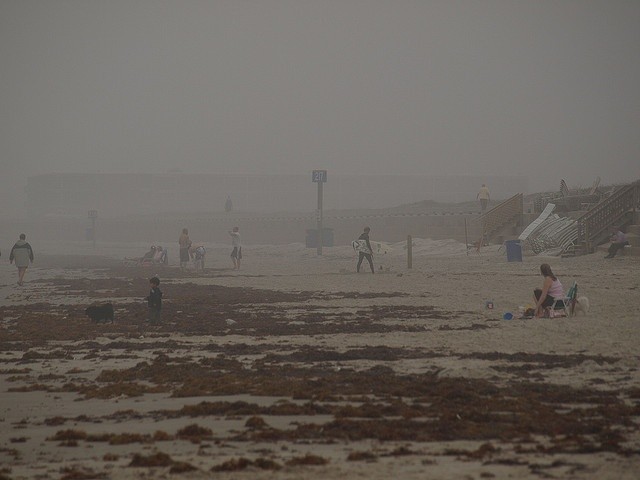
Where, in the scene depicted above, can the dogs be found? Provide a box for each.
[84,303,114,324]
[572,297,589,317]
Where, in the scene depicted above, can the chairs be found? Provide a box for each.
[547,281,577,320]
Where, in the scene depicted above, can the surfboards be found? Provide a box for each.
[351,239,392,257]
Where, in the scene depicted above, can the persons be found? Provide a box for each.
[476,184,491,213]
[224,195,232,213]
[184,240,206,276]
[144,277,162,326]
[532,263,566,318]
[179,228,192,268]
[356,226,375,274]
[228,226,243,270]
[143,246,163,267]
[140,246,156,265]
[604,225,628,258]
[10,234,34,286]
